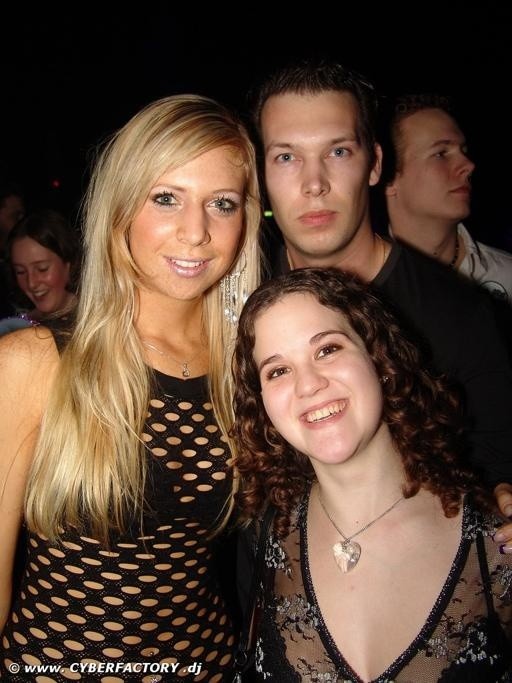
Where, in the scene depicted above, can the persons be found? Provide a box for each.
[228,267,512,683]
[0,93,512,683]
[0,182,27,249]
[375,93,511,298]
[250,60,512,557]
[0,214,79,328]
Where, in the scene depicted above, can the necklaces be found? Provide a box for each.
[315,490,406,574]
[449,235,459,269]
[139,339,208,377]
